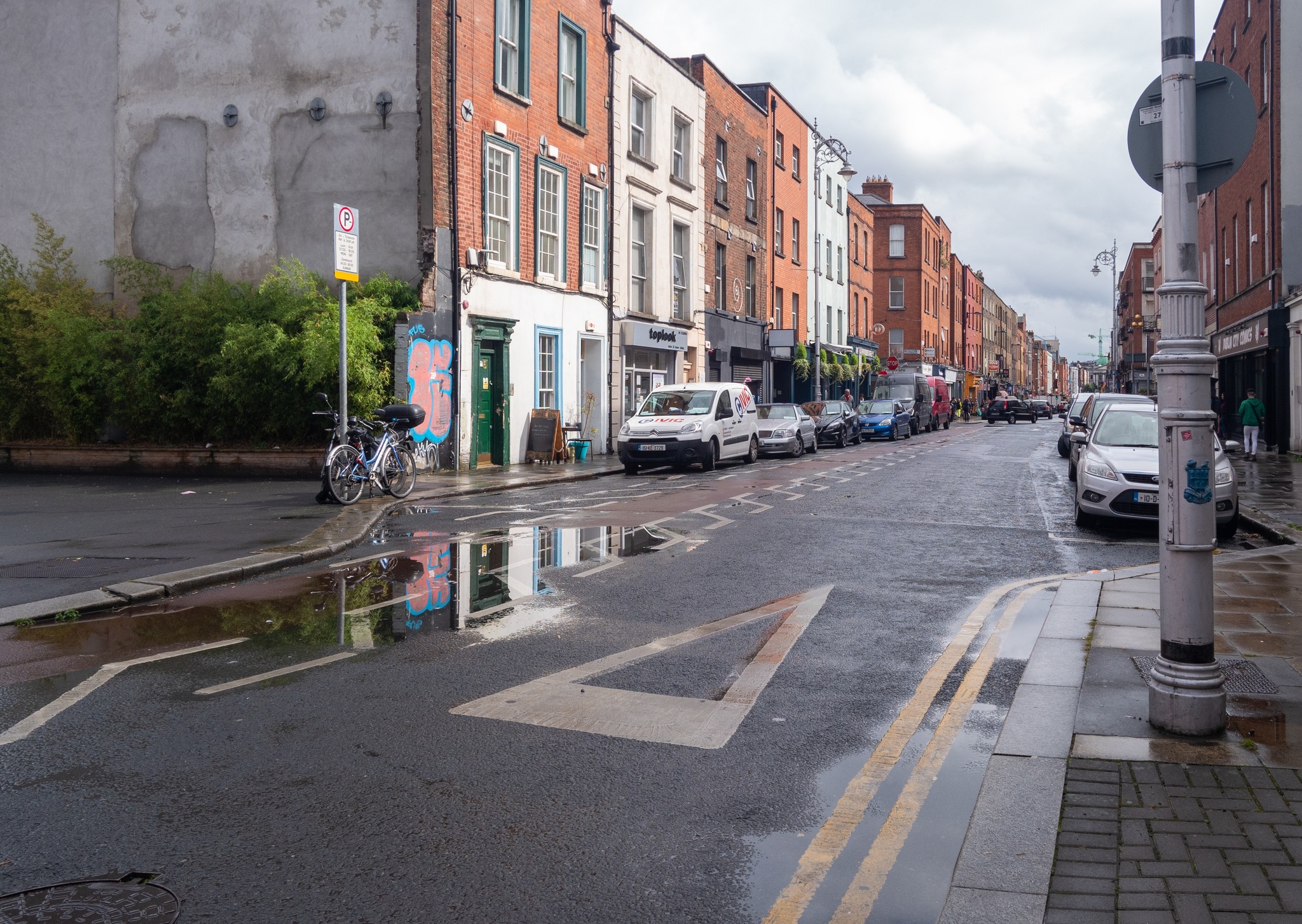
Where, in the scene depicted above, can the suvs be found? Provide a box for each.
[982,396,1057,424]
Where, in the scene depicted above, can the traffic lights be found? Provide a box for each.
[1056,395,1059,399]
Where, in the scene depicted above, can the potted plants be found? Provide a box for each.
[570,388,601,459]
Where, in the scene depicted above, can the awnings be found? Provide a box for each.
[996,390,1008,396]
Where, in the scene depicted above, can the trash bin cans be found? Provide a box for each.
[950,403,954,422]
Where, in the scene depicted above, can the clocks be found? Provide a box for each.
[1157,318,1161,329]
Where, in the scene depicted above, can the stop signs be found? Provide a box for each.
[877,370,888,378]
[886,356,898,371]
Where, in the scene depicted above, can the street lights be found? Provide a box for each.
[1050,388,1053,404]
[1090,239,1118,396]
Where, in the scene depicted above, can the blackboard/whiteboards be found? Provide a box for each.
[526,416,557,454]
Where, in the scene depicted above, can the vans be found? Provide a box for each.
[926,376,954,431]
[617,373,759,475]
[873,373,933,435]
[1061,401,1071,413]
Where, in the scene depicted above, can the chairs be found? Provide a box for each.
[786,409,793,415]
[566,422,593,461]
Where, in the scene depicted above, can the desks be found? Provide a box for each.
[562,427,575,462]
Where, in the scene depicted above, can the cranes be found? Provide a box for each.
[1077,328,1111,358]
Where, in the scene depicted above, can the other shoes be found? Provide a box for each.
[1220,434,1227,440]
[1244,452,1250,459]
[1252,455,1257,461]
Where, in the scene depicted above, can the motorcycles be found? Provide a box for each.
[312,392,426,502]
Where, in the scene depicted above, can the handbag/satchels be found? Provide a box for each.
[1258,422,1263,431]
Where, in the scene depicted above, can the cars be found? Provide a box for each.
[799,400,862,448]
[1069,394,1158,482]
[1070,392,1078,405]
[1057,392,1094,458]
[754,403,818,458]
[855,400,912,441]
[1075,401,1240,540]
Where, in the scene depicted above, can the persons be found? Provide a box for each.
[1057,404,1060,414]
[1238,388,1265,461]
[949,396,991,422]
[1005,396,1008,399]
[1060,402,1064,414]
[859,394,866,404]
[1211,387,1231,440]
[840,389,855,411]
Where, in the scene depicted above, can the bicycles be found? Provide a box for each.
[326,418,416,505]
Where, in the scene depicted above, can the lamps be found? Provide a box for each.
[1224,331,1230,335]
[1232,328,1237,332]
[1240,324,1245,329]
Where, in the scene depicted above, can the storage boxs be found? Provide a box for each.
[379,404,426,429]
[378,562,424,584]
